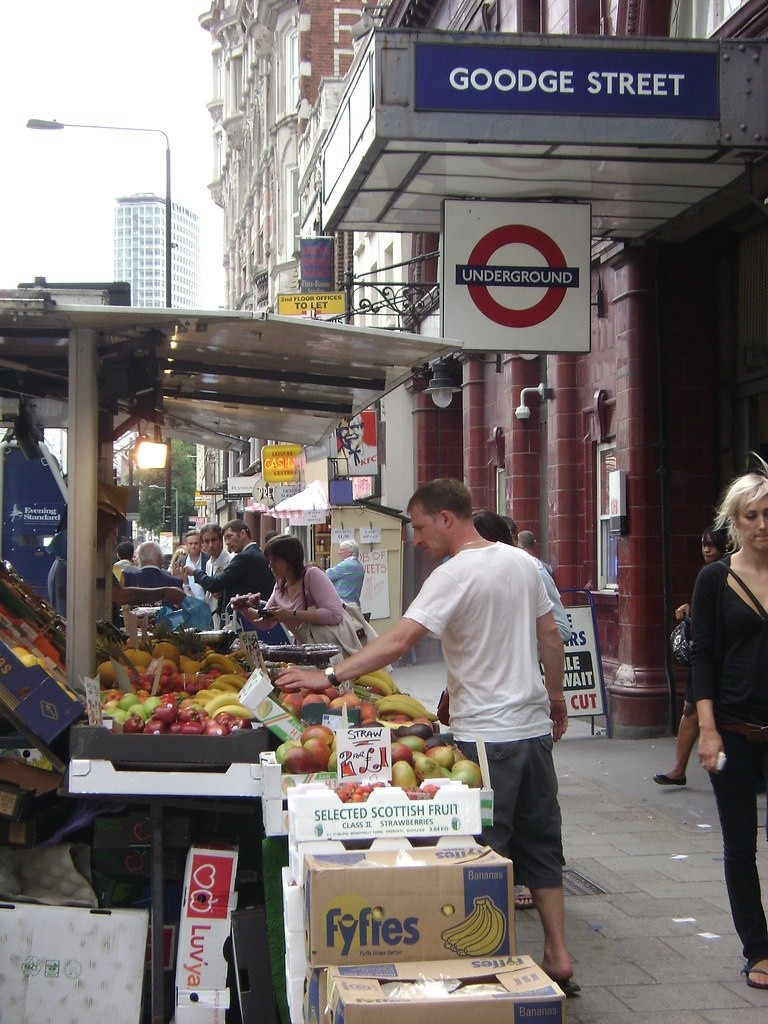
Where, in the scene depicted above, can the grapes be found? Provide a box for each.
[29,754,51,770]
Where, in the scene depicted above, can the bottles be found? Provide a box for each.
[316,524,330,571]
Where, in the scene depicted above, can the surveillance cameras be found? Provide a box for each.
[514,406,531,421]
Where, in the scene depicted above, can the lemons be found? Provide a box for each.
[11,646,77,700]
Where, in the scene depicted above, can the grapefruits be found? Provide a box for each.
[95,641,200,685]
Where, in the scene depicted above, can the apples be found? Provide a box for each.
[102,686,188,721]
[122,704,254,737]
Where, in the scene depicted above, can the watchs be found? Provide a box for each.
[324,666,341,687]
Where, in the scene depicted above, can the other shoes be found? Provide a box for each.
[654,774,686,785]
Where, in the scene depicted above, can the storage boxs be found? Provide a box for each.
[0,557,567,1024]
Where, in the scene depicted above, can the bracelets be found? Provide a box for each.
[548,697,565,702]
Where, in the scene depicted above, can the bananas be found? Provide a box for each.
[372,693,438,721]
[357,668,398,695]
[189,654,256,719]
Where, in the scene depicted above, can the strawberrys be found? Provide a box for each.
[333,783,374,803]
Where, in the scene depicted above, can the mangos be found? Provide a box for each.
[275,724,482,788]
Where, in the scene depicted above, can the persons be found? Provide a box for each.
[114,542,139,567]
[688,473,768,989]
[326,539,364,610]
[185,520,276,602]
[500,515,553,576]
[200,524,233,576]
[122,541,183,590]
[653,528,731,786]
[437,510,571,909]
[237,534,342,645]
[276,477,581,992]
[166,530,210,600]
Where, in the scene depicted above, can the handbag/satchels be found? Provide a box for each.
[156,595,214,632]
[437,687,450,726]
[293,570,366,659]
[669,615,692,664]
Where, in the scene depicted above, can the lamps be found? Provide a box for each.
[422,350,500,409]
[350,3,388,43]
[14,406,43,461]
[135,433,168,470]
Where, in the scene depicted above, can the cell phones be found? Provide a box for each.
[717,751,727,770]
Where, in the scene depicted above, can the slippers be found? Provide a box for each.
[559,981,581,993]
[514,885,535,907]
[746,961,768,988]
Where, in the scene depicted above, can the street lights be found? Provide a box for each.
[148,485,178,550]
[26,118,173,570]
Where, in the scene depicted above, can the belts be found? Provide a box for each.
[717,722,768,742]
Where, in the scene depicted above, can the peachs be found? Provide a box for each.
[274,673,433,730]
